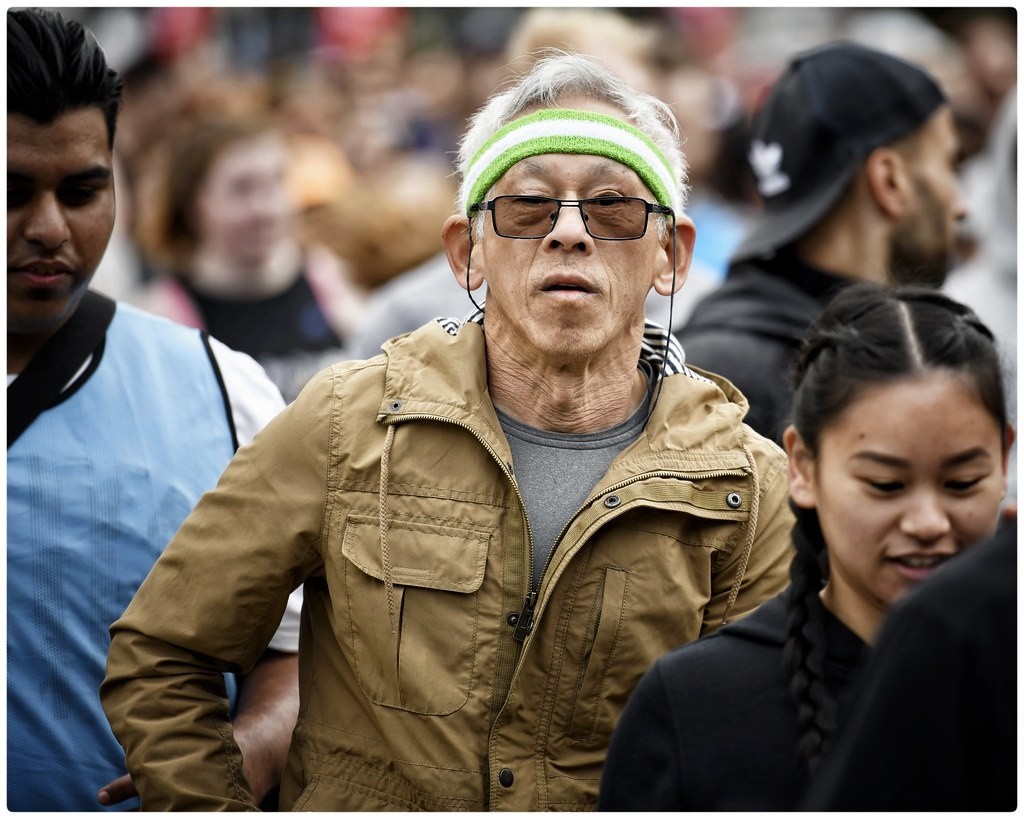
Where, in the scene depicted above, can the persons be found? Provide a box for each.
[8,8,304,812]
[805,517,1017,812]
[597,284,1017,811]
[109,8,1017,513]
[660,37,966,450]
[99,53,795,812]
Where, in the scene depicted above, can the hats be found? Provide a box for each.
[729,42,946,265]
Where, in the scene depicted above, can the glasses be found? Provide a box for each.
[469,194,672,241]
